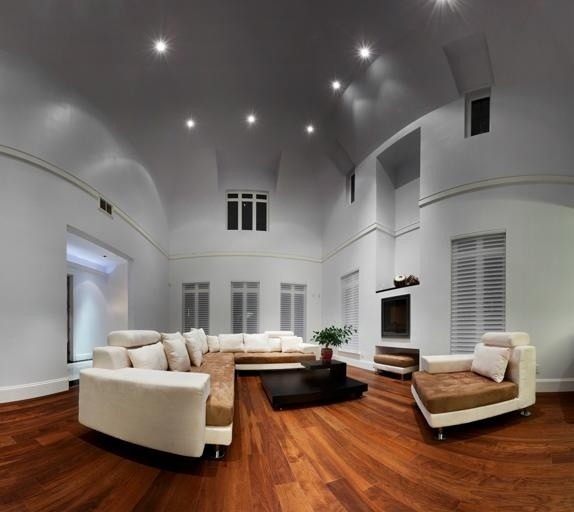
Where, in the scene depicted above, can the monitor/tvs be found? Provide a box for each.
[381,293,411,338]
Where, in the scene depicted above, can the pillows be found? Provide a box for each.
[470,346,510,383]
[126,341,168,370]
[160,331,185,344]
[207,335,220,353]
[185,336,202,367]
[108,329,161,347]
[164,336,189,372]
[263,330,293,338]
[198,328,208,354]
[244,334,268,353]
[182,327,203,354]
[218,333,245,353]
[280,336,300,352]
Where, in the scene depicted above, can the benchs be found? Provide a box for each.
[372,354,419,381]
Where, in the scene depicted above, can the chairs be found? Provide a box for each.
[410,332,537,440]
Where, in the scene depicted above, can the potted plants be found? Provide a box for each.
[313,323,357,361]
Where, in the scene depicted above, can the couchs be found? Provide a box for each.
[77,330,234,459]
[232,334,314,377]
